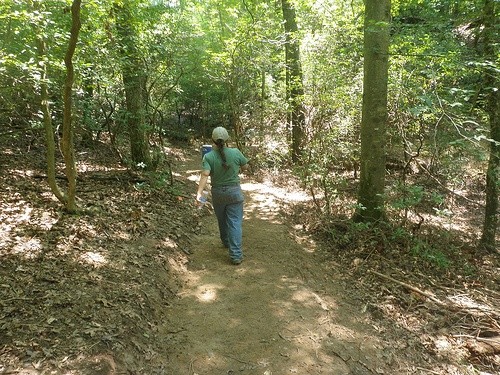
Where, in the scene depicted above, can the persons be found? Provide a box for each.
[194,127,249,265]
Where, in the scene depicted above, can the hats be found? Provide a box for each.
[212,127,229,142]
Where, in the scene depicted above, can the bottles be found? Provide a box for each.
[197,191,208,209]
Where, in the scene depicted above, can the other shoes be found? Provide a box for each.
[232,256,243,264]
[225,243,230,248]
